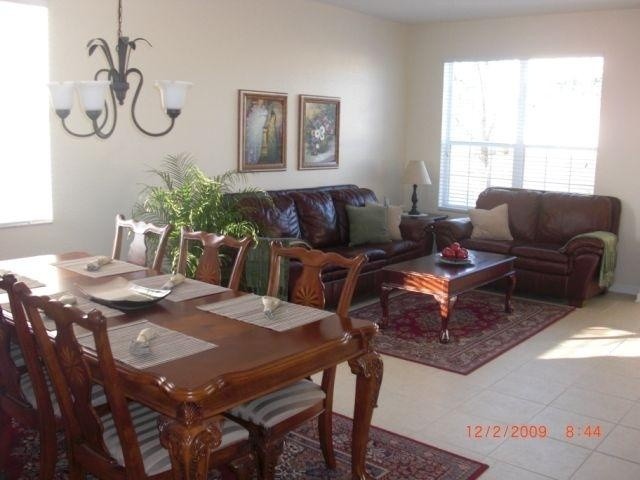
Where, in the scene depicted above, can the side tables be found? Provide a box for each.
[398,208,448,241]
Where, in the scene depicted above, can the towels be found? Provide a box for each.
[132,328,159,356]
[262,294,281,320]
[158,274,190,294]
[85,255,115,273]
[45,293,77,307]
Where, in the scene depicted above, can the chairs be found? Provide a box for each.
[110,210,174,270]
[2,275,125,478]
[14,282,254,478]
[176,224,253,291]
[222,241,370,480]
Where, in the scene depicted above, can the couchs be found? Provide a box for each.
[436,185,620,306]
[195,181,432,310]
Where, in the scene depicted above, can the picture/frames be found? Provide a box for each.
[298,95,342,172]
[239,88,289,174]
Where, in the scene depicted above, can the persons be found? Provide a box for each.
[265,110,277,163]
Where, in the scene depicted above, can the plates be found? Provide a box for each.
[71,275,173,314]
[438,254,476,267]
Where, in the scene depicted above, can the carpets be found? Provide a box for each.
[332,288,578,377]
[0,403,491,480]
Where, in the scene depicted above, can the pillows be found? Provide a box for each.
[346,205,390,246]
[371,200,407,239]
[467,203,514,242]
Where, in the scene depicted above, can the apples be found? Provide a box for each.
[443,241,469,260]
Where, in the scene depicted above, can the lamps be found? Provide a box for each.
[48,0,186,141]
[399,160,432,216]
